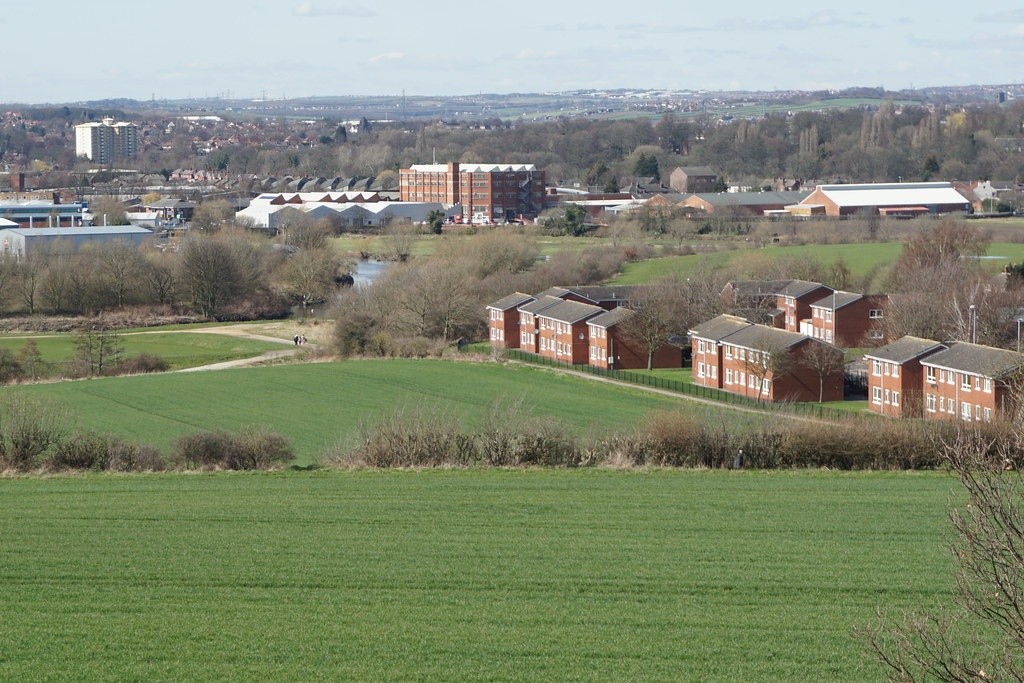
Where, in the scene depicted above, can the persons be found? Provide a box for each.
[293,333,306,346]
